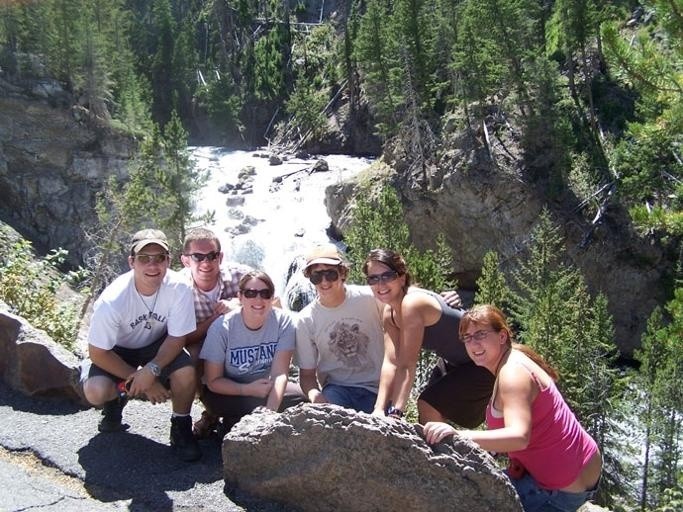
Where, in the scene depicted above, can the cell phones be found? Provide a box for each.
[125,378,133,392]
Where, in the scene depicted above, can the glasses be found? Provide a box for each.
[240,286,274,301]
[182,249,220,262]
[459,326,500,343]
[363,269,401,286]
[305,267,344,286]
[131,252,168,265]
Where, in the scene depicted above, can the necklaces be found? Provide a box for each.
[495,349,508,375]
[136,286,160,317]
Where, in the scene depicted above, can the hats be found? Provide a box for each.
[296,241,352,280]
[127,226,171,255]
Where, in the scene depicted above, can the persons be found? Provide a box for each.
[176,228,282,437]
[295,244,463,415]
[198,271,311,440]
[422,304,602,512]
[362,250,495,429]
[79,229,199,461]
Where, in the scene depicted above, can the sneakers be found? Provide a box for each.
[96,393,132,433]
[193,410,220,440]
[167,414,204,465]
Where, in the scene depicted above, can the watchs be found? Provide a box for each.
[388,406,403,417]
[147,362,162,378]
